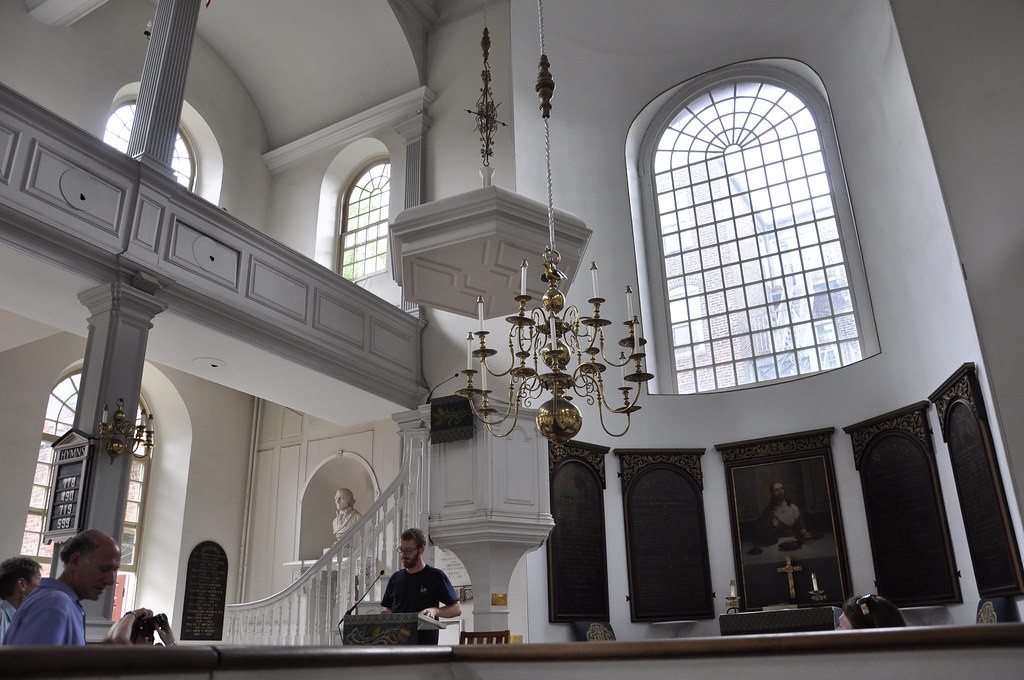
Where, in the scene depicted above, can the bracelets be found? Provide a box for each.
[166,640,178,647]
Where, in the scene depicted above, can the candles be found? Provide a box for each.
[808,572,828,607]
[725,580,743,615]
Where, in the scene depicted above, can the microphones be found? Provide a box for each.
[339,570,385,625]
[425,373,459,403]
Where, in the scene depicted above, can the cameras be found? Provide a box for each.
[133,614,167,637]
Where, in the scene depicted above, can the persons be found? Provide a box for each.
[0,556,43,647]
[372,528,461,620]
[103,608,181,648]
[829,595,903,632]
[331,488,365,546]
[3,528,120,648]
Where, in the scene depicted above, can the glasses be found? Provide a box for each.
[396,545,422,554]
[856,593,879,628]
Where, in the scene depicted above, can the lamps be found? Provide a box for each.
[454,246,655,459]
[98,397,156,466]
